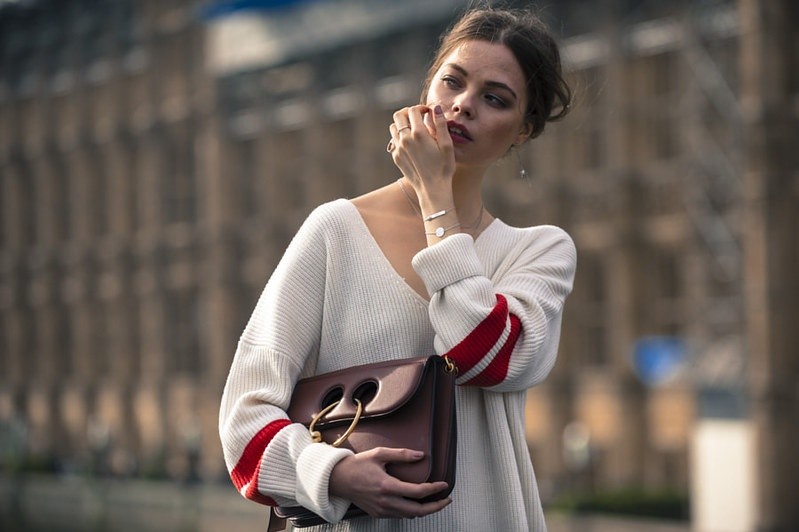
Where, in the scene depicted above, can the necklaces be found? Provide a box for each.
[397,178,482,238]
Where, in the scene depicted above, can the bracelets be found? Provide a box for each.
[425,223,461,237]
[423,208,456,223]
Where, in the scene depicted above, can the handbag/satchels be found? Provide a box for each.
[267,354,459,532]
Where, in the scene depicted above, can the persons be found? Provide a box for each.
[216,3,588,532]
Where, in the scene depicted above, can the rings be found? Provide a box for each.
[397,125,411,135]
[387,139,395,152]
